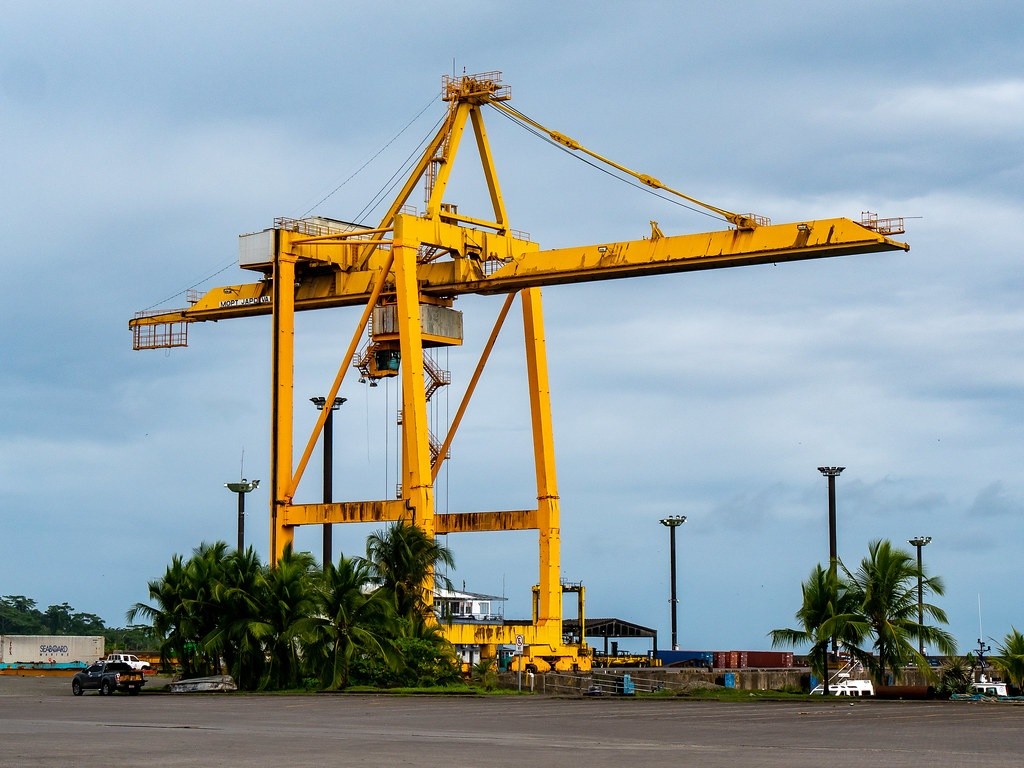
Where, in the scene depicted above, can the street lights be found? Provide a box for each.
[659,515,689,651]
[225,454,259,555]
[907,536,933,654]
[308,396,348,572]
[818,466,848,650]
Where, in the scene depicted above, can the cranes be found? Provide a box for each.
[130,62,912,668]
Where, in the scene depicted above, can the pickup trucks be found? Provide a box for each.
[71,662,146,696]
[95,653,151,671]
